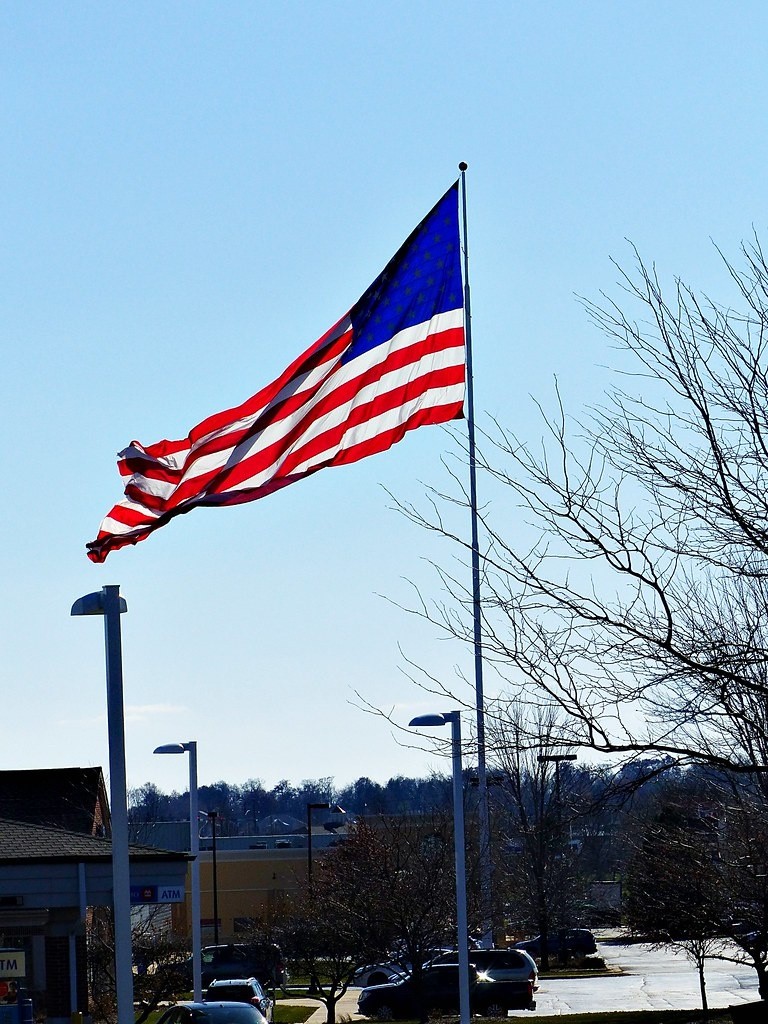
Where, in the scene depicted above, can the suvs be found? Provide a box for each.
[154,942,287,990]
[512,929,598,963]
[388,948,541,991]
[206,976,275,1024]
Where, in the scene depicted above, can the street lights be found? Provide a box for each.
[73,582,138,1024]
[537,754,579,927]
[408,710,470,1024]
[307,803,331,907]
[151,742,202,1004]
[208,811,219,944]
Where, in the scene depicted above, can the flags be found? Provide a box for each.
[84,178,469,563]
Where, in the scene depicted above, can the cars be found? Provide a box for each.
[353,946,454,991]
[274,839,293,849]
[252,841,269,849]
[155,1001,269,1024]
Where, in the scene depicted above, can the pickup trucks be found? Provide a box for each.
[356,963,536,1022]
